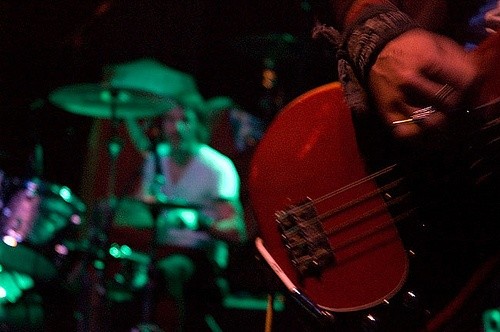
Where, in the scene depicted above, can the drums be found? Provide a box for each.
[104,226,211,301]
[0,179,87,276]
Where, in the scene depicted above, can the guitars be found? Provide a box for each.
[249,31,500,323]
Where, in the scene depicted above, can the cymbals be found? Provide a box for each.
[129,194,205,208]
[49,82,169,118]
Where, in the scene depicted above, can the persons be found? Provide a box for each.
[133,96,248,328]
[334,0,499,331]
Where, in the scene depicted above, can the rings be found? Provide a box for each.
[409,105,439,119]
[432,82,456,103]
[389,118,416,127]
